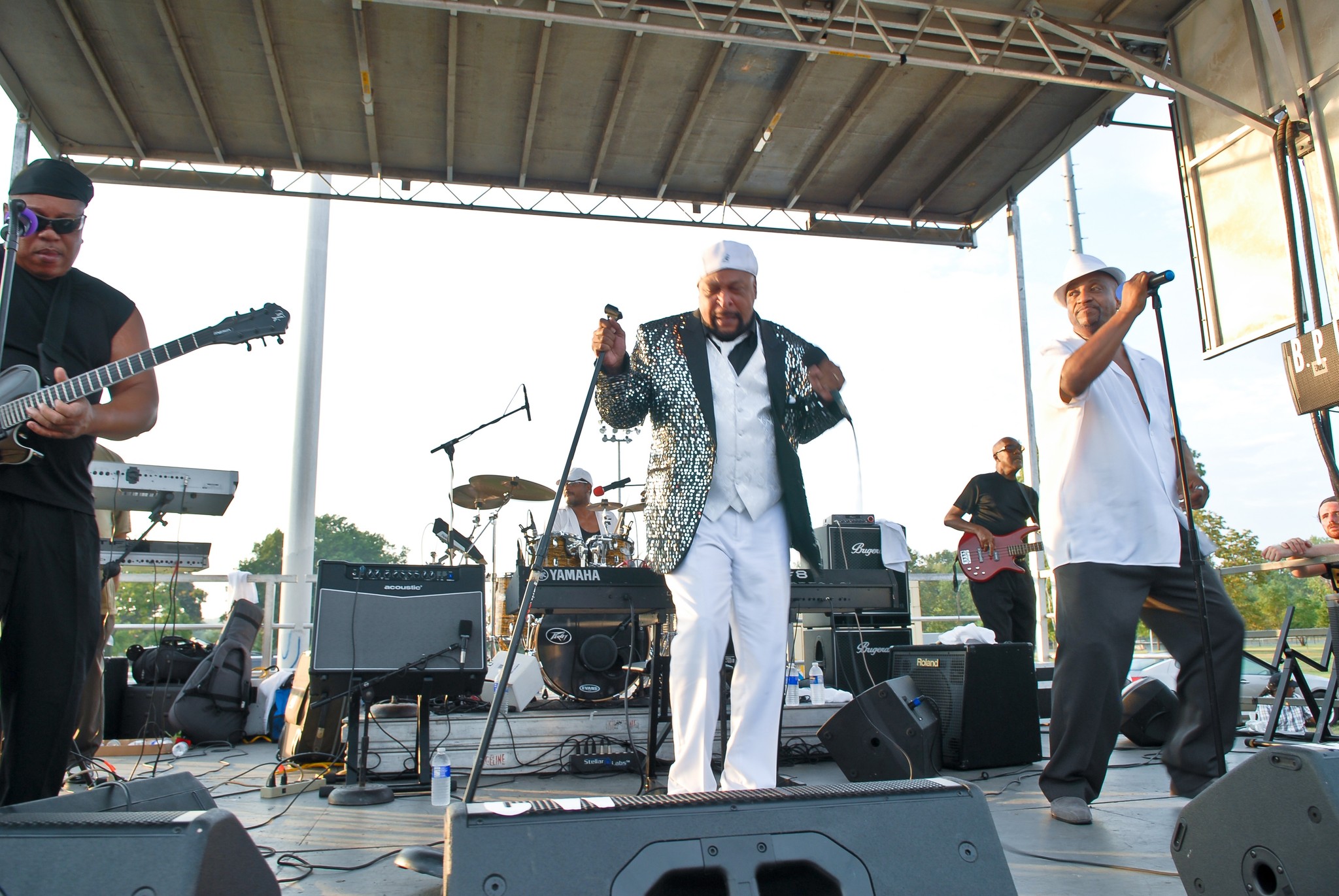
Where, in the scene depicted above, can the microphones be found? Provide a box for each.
[619,510,626,534]
[1116,269,1174,302]
[458,618,473,670]
[594,477,630,497]
[4,208,38,237]
[524,384,531,421]
[530,512,537,536]
[517,540,526,559]
[803,346,852,420]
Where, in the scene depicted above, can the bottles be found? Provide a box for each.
[128,737,173,746]
[106,739,121,746]
[494,664,509,714]
[809,662,826,705]
[431,747,451,806]
[784,663,799,706]
[172,742,189,757]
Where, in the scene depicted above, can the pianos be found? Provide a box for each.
[87,458,240,588]
[505,565,900,778]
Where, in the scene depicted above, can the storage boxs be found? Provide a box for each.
[480,651,544,712]
[94,738,174,757]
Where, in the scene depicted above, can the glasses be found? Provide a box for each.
[564,481,588,488]
[36,213,87,235]
[995,444,1026,454]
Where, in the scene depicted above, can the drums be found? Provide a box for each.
[585,533,635,567]
[527,612,651,703]
[487,572,530,645]
[528,532,584,567]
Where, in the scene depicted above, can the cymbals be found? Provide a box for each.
[586,502,623,512]
[468,475,556,501]
[448,484,510,510]
[617,503,645,512]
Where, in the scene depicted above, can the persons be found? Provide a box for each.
[1255,496,1339,733]
[545,467,620,543]
[1034,254,1246,821]
[944,437,1040,648]
[1,158,160,804]
[593,239,845,793]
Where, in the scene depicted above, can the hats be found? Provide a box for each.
[1269,672,1299,690]
[695,240,757,291]
[556,468,593,487]
[8,159,94,207]
[1051,254,1126,309]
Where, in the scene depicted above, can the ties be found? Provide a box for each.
[708,329,750,360]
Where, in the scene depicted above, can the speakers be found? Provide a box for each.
[314,559,488,697]
[0,771,219,813]
[442,777,1018,896]
[798,524,914,630]
[800,628,913,698]
[1168,740,1339,896]
[887,642,1042,772]
[815,675,941,784]
[0,808,281,896]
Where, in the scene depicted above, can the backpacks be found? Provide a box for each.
[127,635,214,684]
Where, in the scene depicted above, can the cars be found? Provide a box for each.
[1125,653,1338,728]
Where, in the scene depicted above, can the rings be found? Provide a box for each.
[1195,485,1204,491]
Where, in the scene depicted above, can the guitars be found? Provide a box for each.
[0,302,291,466]
[957,525,1044,582]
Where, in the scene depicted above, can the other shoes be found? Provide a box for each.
[1051,796,1096,824]
[68,762,93,783]
[1172,776,1222,799]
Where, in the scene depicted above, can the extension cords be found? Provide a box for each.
[260,776,327,798]
